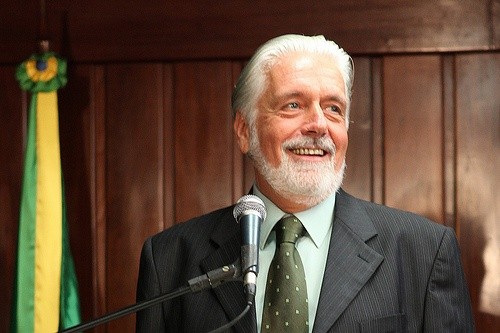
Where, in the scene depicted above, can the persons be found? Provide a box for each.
[134,34,475,333]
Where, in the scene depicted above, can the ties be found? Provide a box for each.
[261,216,310,333]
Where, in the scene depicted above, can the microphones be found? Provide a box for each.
[233,195,267,306]
